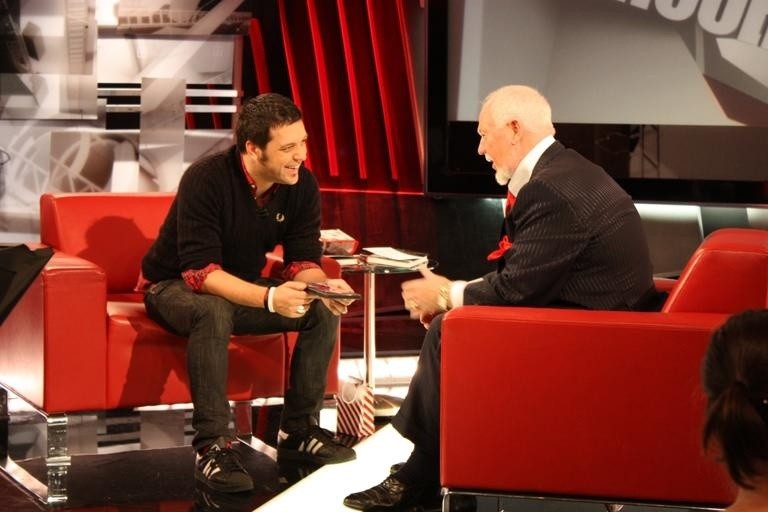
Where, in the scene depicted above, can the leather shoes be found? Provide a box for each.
[344,463,477,511]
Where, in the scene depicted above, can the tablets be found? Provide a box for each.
[306,282,361,300]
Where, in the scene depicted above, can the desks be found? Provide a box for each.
[318,245,437,419]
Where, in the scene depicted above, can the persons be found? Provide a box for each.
[344,84,658,512]
[132,93,356,494]
[702,309,768,512]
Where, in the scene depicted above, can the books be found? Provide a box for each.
[322,255,359,266]
[358,253,428,269]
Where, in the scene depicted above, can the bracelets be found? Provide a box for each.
[436,285,449,314]
[263,286,274,314]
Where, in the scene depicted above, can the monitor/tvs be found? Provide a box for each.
[426,0,768,209]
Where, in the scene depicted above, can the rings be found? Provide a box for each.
[408,301,418,311]
[297,305,305,314]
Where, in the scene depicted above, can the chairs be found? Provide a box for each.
[1,189,340,503]
[433,228,768,511]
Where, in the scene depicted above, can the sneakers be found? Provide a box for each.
[192,435,254,494]
[277,424,355,463]
[194,478,245,512]
